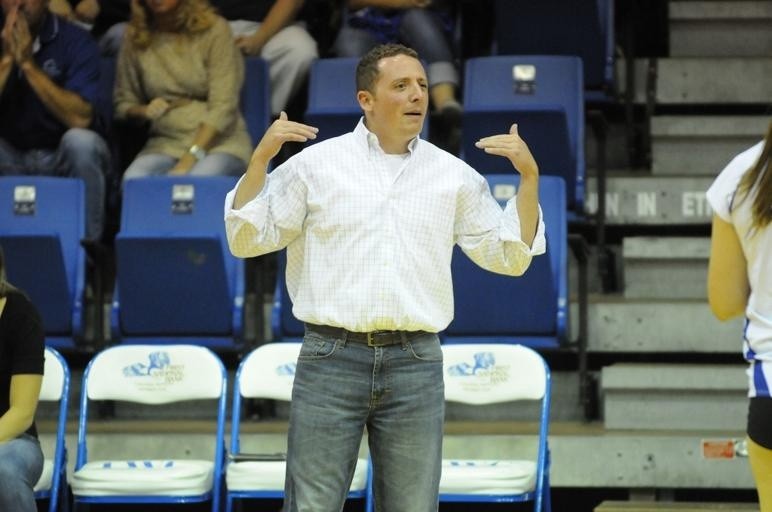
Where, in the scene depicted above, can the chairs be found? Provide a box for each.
[0,0,669,510]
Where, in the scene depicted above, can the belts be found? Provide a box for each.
[307,323,427,347]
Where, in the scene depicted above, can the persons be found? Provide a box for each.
[0,250,45,512]
[705,116,772,512]
[225,44,547,512]
